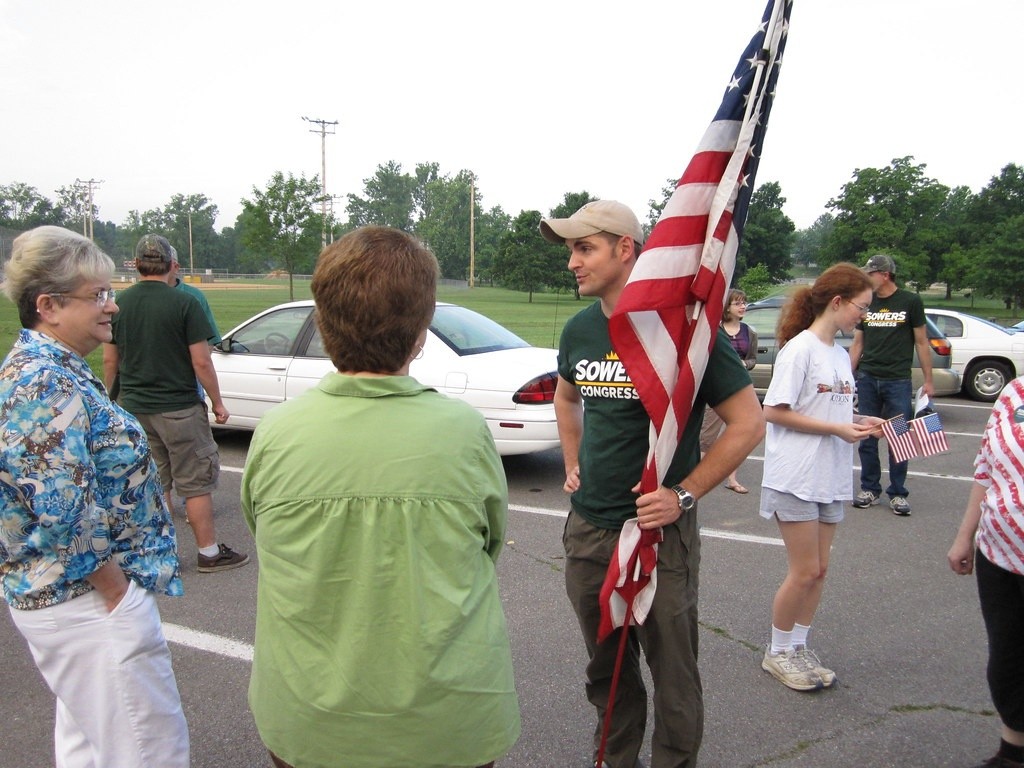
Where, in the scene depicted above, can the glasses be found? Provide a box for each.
[845,299,870,313]
[37,290,116,313]
[730,302,746,307]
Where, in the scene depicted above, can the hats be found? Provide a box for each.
[861,255,895,275]
[540,200,644,246]
[136,234,172,264]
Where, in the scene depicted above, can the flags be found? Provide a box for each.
[880,415,917,464]
[591,0,793,642]
[912,414,948,457]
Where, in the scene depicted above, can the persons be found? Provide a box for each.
[759,264,888,690]
[0,224,191,768]
[538,201,766,768]
[698,289,758,493]
[848,255,933,516]
[103,233,248,573]
[239,226,522,768]
[164,246,222,524]
[946,374,1024,768]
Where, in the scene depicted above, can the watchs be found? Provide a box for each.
[671,484,694,511]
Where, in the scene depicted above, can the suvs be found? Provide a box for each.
[741,297,962,417]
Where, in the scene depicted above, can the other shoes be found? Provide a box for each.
[198,544,249,572]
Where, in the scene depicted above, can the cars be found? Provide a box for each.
[199,294,586,458]
[922,308,1024,404]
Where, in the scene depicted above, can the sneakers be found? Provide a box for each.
[796,644,838,686]
[890,496,911,515]
[852,489,879,507]
[762,643,823,690]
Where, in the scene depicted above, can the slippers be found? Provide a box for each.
[725,484,748,493]
